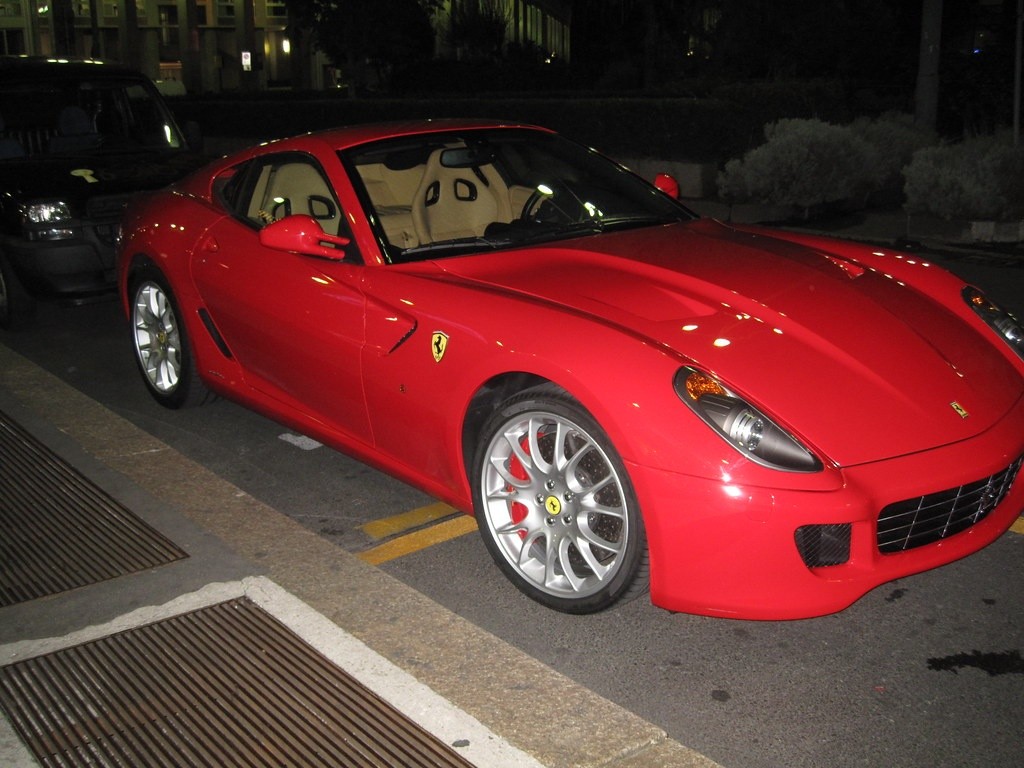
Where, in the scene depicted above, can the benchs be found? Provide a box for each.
[247,163,438,245]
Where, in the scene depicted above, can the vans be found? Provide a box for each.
[0,52,206,333]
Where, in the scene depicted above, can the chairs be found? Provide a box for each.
[411,149,512,244]
[260,163,342,249]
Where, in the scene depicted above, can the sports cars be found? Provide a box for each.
[114,115,1023,622]
